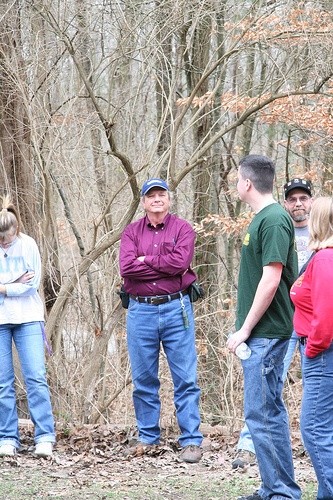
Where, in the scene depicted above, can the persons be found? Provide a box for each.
[289,197,332,500]
[230,178,317,469]
[225,154,301,500]
[0,194,56,456]
[119,177,202,463]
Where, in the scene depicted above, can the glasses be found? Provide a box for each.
[287,196,310,204]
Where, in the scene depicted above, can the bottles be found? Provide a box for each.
[227,334,251,360]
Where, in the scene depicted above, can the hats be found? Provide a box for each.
[283,178,313,198]
[141,178,168,195]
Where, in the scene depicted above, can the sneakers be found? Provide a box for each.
[124,443,159,457]
[35,442,53,456]
[232,450,256,468]
[0,443,15,457]
[179,445,202,462]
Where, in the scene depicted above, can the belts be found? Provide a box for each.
[299,337,305,345]
[129,291,186,306]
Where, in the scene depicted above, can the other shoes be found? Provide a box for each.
[237,490,262,500]
